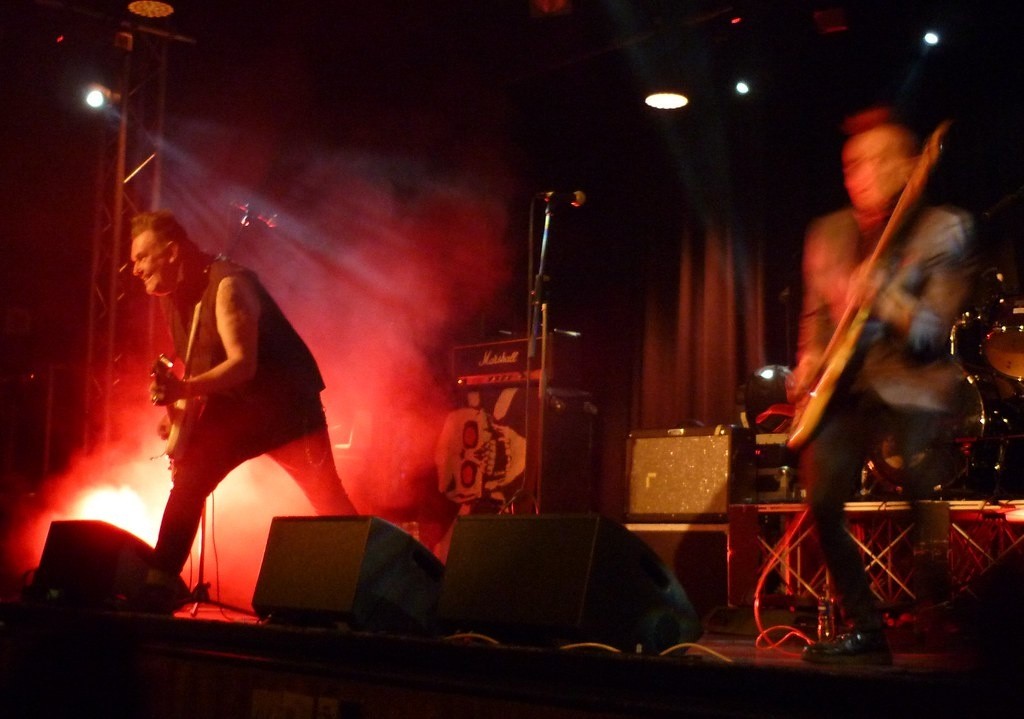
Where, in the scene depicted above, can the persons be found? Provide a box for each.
[788,111,977,665]
[130,210,357,573]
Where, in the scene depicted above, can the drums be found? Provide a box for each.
[861,353,988,500]
[974,285,1024,381]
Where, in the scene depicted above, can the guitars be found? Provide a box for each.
[148,354,196,463]
[758,122,946,448]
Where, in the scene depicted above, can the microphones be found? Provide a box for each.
[537,191,586,206]
[233,200,279,227]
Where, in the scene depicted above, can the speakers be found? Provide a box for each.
[31,520,191,616]
[625,424,757,524]
[455,387,595,516]
[953,533,1024,672]
[253,515,447,637]
[436,513,706,658]
